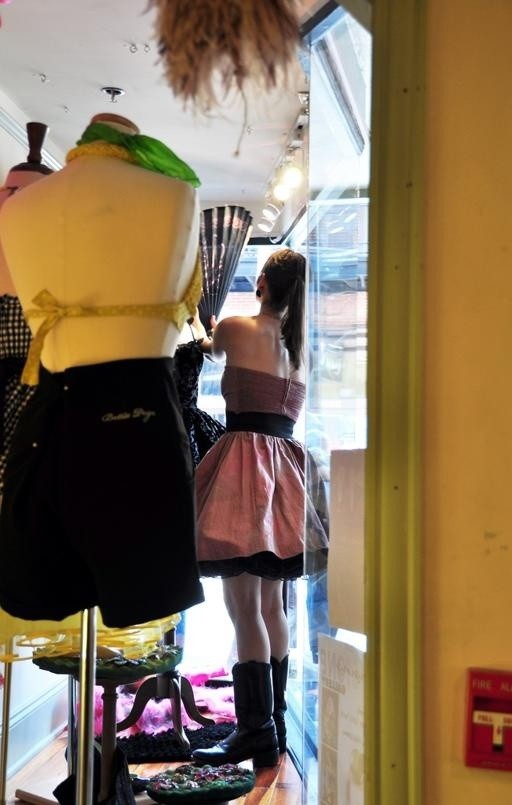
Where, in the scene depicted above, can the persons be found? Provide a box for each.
[191,247,326,769]
[1,110,206,630]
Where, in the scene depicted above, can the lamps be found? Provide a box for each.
[258,111,309,233]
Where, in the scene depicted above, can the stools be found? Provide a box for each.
[33,640,183,804]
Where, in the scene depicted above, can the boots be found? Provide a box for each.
[191,654,289,768]
